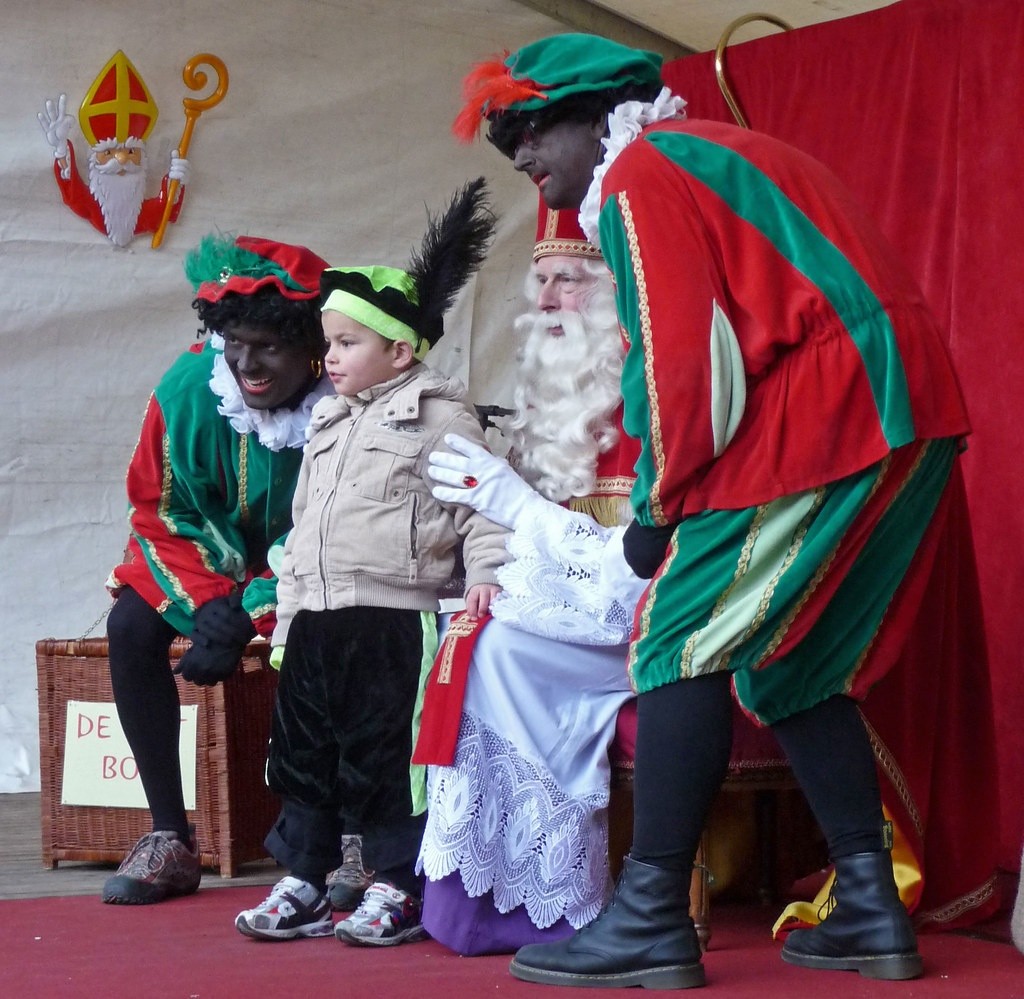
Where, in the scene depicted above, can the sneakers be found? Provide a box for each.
[336,880,431,946]
[102,820,201,904]
[326,835,372,909]
[234,875,335,940]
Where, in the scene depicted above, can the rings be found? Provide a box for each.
[464,475,478,487]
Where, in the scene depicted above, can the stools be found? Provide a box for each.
[611,697,796,953]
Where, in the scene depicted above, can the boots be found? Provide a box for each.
[781,847,922,980]
[511,856,705,992]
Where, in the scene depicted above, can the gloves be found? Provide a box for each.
[428,434,556,530]
[190,594,254,649]
[174,644,247,687]
[623,517,681,581]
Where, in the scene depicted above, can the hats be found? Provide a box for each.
[190,234,331,300]
[533,190,605,261]
[454,32,665,139]
[320,265,444,364]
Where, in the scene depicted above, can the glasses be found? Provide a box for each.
[485,102,593,158]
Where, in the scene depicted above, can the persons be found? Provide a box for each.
[102,238,383,907]
[235,266,517,944]
[413,192,653,956]
[483,34,974,987]
